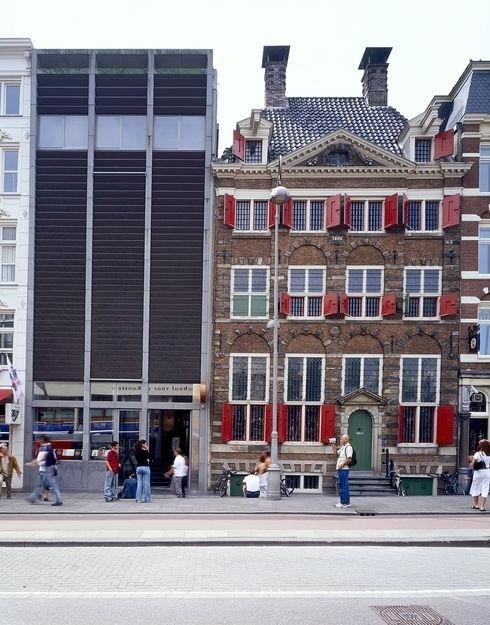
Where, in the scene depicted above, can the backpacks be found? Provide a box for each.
[40,448,56,466]
[345,444,356,468]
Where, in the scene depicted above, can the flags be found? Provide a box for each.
[6,356,23,404]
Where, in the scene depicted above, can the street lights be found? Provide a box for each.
[266,155,289,502]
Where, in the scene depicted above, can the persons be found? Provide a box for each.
[242,468,260,498]
[24,435,63,506]
[263,449,271,466]
[330,434,353,508]
[468,439,490,511]
[0,442,23,499]
[164,448,189,498]
[105,440,152,503]
[256,456,266,474]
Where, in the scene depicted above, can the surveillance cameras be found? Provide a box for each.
[470,386,479,394]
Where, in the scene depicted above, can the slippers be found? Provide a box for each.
[472,505,486,511]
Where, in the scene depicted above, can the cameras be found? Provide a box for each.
[328,438,337,444]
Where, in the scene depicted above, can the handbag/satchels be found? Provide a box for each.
[473,461,486,470]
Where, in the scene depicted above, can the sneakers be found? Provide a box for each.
[335,502,350,509]
[41,496,63,507]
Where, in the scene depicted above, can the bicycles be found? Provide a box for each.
[441,470,465,496]
[214,464,237,497]
[389,468,406,496]
[280,478,294,497]
